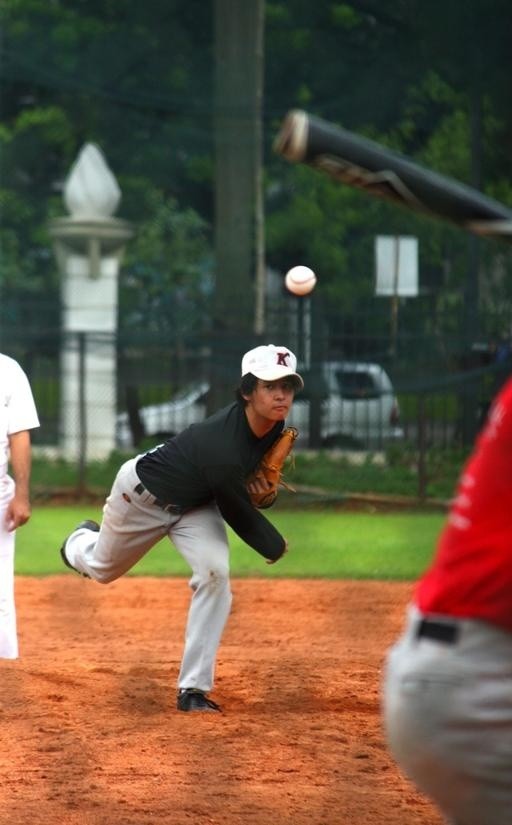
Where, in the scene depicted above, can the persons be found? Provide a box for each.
[377,377,510,822]
[62,346,304,712]
[0,352,41,661]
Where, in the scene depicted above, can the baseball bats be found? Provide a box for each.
[275,110,511,248]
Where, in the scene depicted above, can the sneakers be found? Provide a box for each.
[177,688,221,712]
[60,520,100,579]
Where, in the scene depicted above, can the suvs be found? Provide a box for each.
[117,360,405,451]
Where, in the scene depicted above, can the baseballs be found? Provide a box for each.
[286,265,318,296]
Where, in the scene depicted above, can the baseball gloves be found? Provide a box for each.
[245,427,297,511]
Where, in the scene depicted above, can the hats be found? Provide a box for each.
[241,343,304,390]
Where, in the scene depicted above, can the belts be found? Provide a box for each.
[135,483,188,515]
[416,620,458,643]
